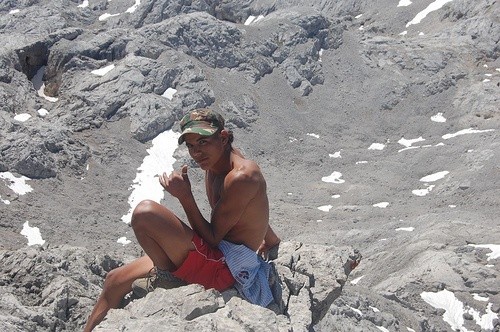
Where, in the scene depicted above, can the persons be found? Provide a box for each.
[83,105,282,332]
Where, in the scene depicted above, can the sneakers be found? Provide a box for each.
[130,272,187,300]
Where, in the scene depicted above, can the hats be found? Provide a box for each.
[178,108,226,145]
